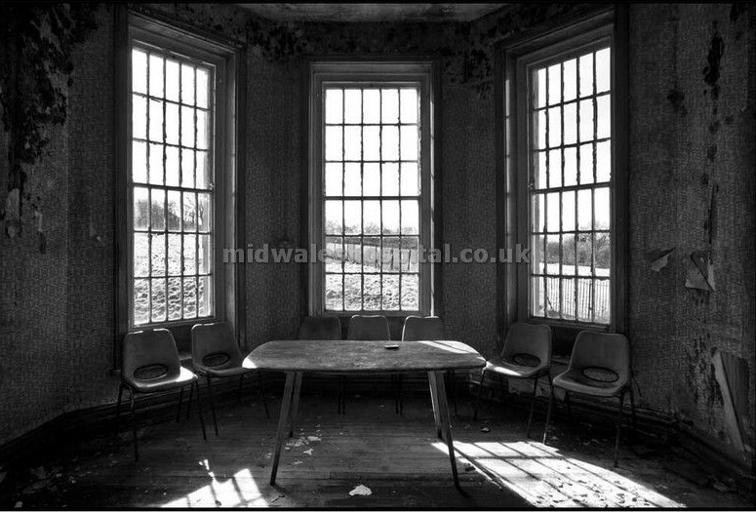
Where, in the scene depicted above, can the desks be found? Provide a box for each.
[241,340,486,491]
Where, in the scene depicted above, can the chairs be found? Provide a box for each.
[298,315,457,416]
[474,321,636,467]
[112,321,270,462]
